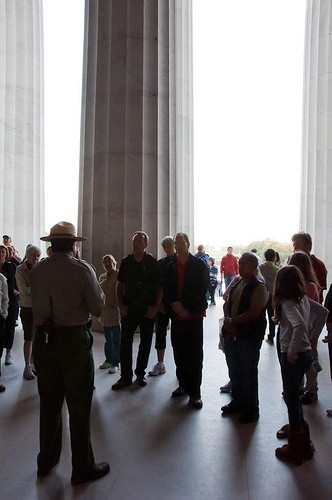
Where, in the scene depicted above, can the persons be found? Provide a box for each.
[0,234,24,392]
[324,283,332,417]
[287,252,325,405]
[259,249,280,345]
[273,265,329,464]
[29,220,110,486]
[221,252,270,424]
[251,248,261,266]
[218,268,266,392]
[148,235,178,376]
[15,245,41,379]
[207,257,218,307]
[162,233,211,408]
[287,233,327,301]
[98,254,120,374]
[220,247,239,290]
[112,231,164,390]
[193,245,209,263]
[275,252,284,269]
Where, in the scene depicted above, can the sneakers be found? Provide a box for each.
[220,381,233,392]
[148,362,166,376]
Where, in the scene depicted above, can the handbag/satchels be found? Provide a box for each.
[219,280,223,297]
[217,317,225,353]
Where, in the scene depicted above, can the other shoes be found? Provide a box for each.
[100,362,111,369]
[37,459,60,479]
[111,379,132,390]
[23,364,35,380]
[277,424,288,438]
[301,392,318,404]
[209,301,216,306]
[326,408,332,415]
[275,440,315,459]
[0,383,6,392]
[5,355,13,365]
[221,399,259,413]
[136,378,146,386]
[266,339,274,345]
[172,387,187,396]
[109,366,119,374]
[190,397,203,409]
[71,461,110,485]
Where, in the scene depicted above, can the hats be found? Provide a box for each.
[40,222,88,242]
[2,233,11,239]
